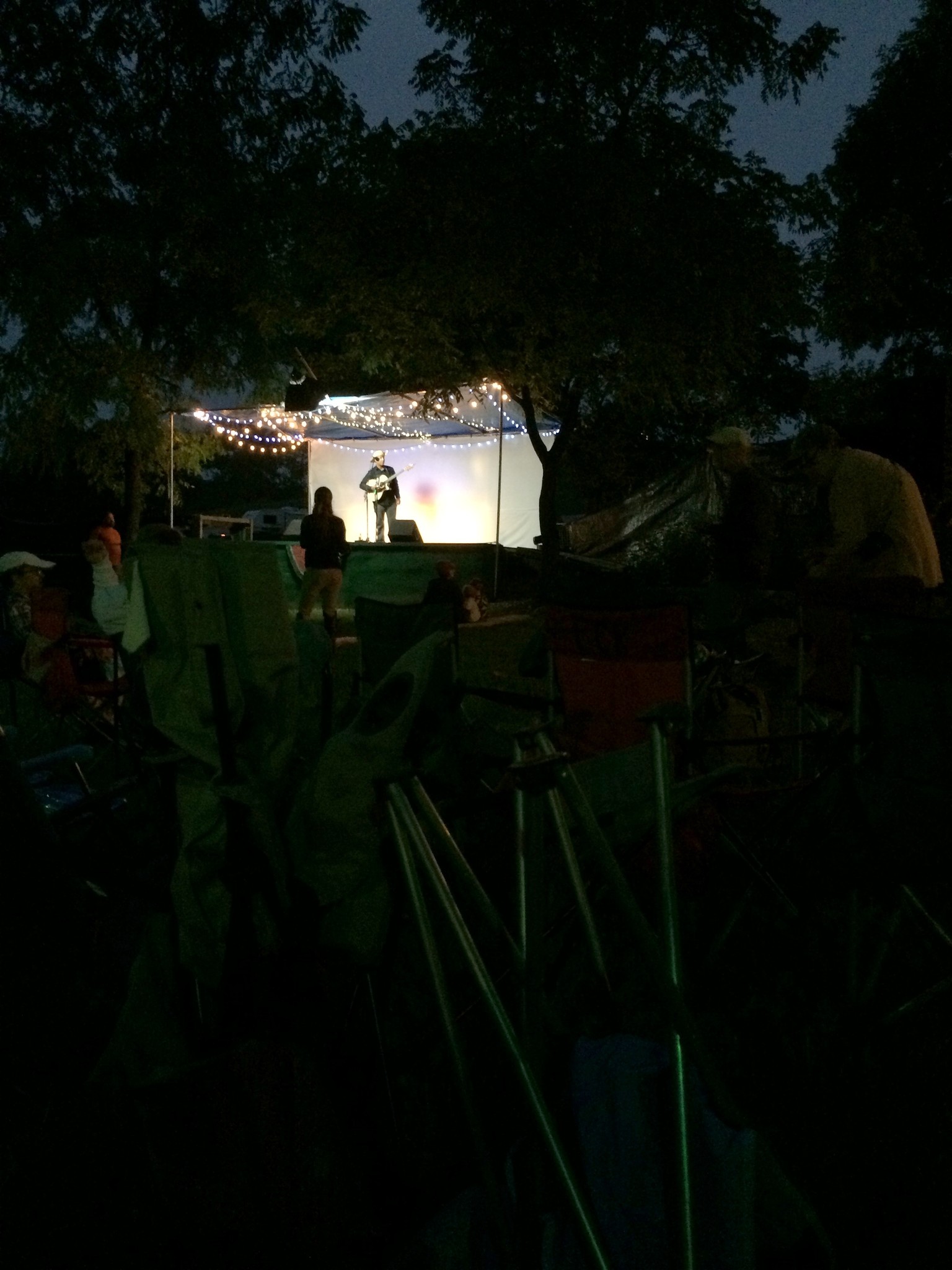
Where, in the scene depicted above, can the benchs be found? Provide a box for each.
[193,514,254,541]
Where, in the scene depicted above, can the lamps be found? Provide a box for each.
[284,367,328,413]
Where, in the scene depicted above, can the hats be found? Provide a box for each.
[370,450,384,462]
[0,551,55,572]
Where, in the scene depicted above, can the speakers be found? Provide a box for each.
[387,523,422,544]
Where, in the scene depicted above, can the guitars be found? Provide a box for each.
[367,462,414,502]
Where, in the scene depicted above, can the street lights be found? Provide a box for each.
[169,408,207,528]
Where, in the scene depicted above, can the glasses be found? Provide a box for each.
[15,569,45,578]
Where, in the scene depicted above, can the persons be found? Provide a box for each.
[792,423,941,589]
[80,525,188,657]
[702,424,797,594]
[360,451,400,544]
[94,511,122,567]
[1,552,66,717]
[294,486,350,639]
[422,560,462,608]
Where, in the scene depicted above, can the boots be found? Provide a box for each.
[323,610,337,656]
